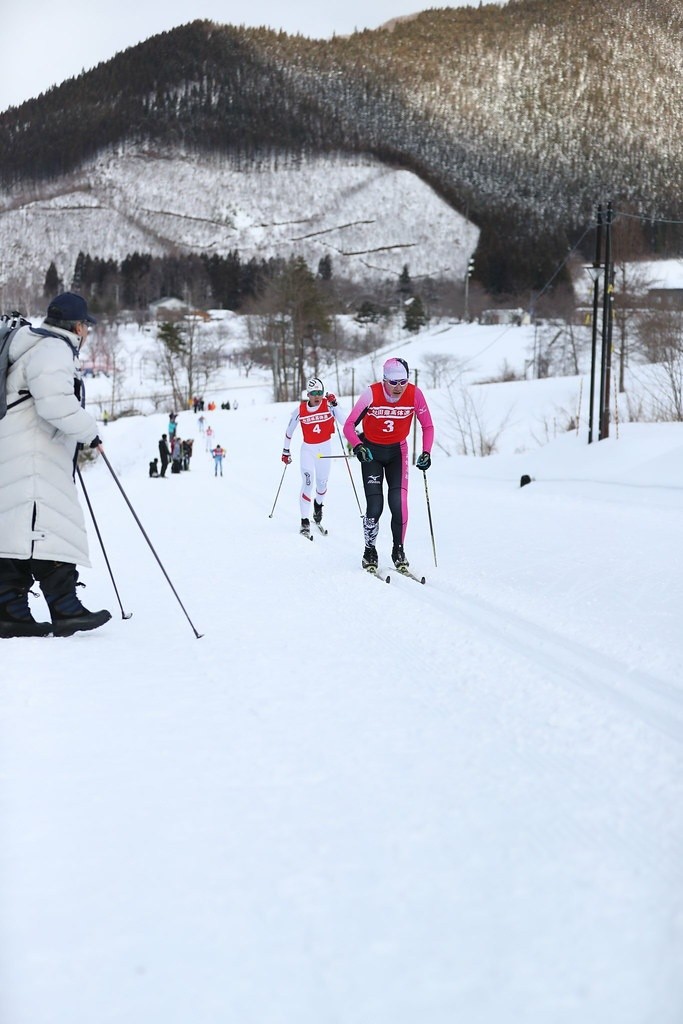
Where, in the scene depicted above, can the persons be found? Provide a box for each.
[198,415,205,433]
[0,291,112,639]
[205,426,214,453]
[211,445,225,477]
[193,396,204,414]
[168,413,178,442]
[207,399,239,410]
[343,357,436,573]
[159,434,171,477]
[103,410,108,425]
[281,378,346,538]
[171,436,194,474]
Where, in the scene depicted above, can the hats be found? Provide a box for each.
[47,292,98,325]
[306,377,324,394]
[383,358,409,383]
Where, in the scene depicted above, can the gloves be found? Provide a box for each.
[281,454,292,464]
[77,443,100,463]
[352,443,373,463]
[415,451,432,470]
[327,394,336,404]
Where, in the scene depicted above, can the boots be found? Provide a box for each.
[38,560,112,637]
[0,572,53,639]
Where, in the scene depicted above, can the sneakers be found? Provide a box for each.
[392,547,410,570]
[362,547,378,572]
[300,518,311,535]
[313,500,324,524]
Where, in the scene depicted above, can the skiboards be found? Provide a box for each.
[303,522,329,542]
[372,566,426,585]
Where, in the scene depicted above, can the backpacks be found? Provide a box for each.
[0,327,76,419]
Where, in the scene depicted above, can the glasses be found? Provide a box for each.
[384,379,409,387]
[75,321,90,329]
[308,390,323,396]
